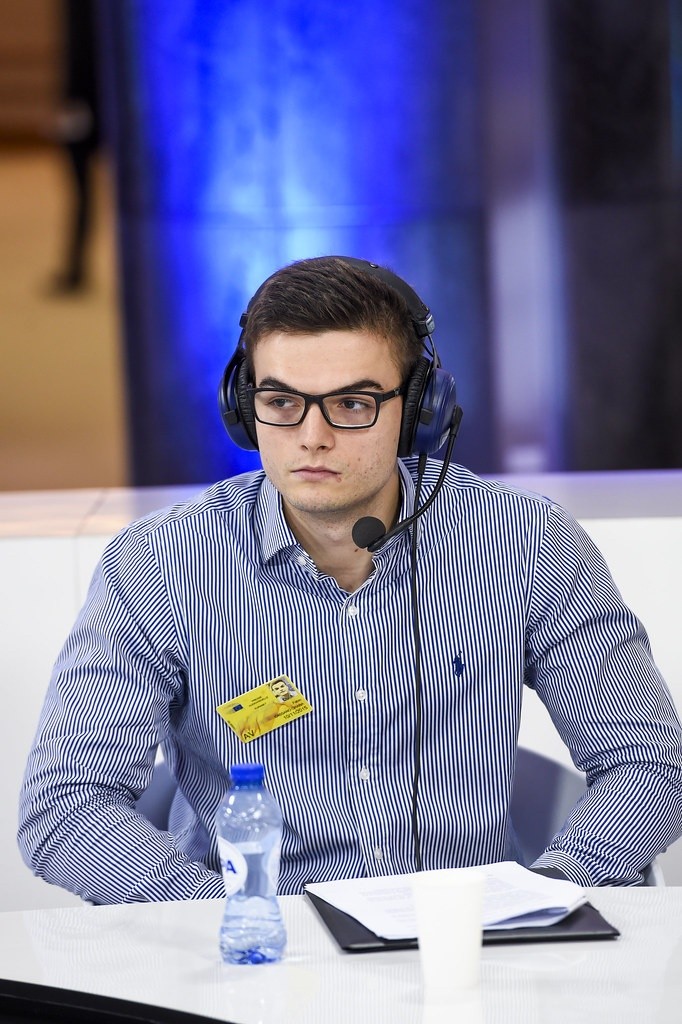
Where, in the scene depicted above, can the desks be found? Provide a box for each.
[0,888,682,1023]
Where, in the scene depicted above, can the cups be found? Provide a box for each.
[415,879,483,967]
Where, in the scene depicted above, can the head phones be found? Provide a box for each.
[217,254,464,458]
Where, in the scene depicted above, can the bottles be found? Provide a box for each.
[216,763,286,966]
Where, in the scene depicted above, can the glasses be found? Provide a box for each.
[246,383,406,430]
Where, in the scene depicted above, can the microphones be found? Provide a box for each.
[352,404,464,552]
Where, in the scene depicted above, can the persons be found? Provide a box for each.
[18,256,681,905]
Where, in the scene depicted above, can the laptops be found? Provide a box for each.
[301,866,620,953]
